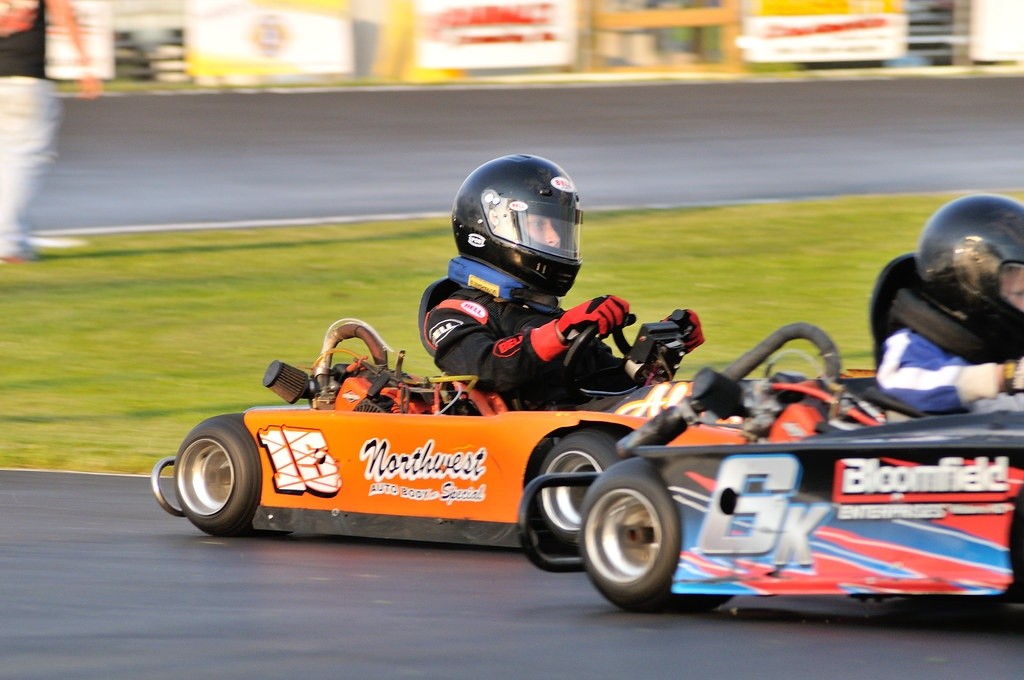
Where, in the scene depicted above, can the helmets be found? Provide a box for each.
[914,194,1024,360]
[450,152,582,296]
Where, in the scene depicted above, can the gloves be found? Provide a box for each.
[674,306,703,356]
[528,296,629,365]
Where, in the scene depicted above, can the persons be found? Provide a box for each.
[876,195,1024,414]
[424,154,704,411]
[0,0,103,261]
